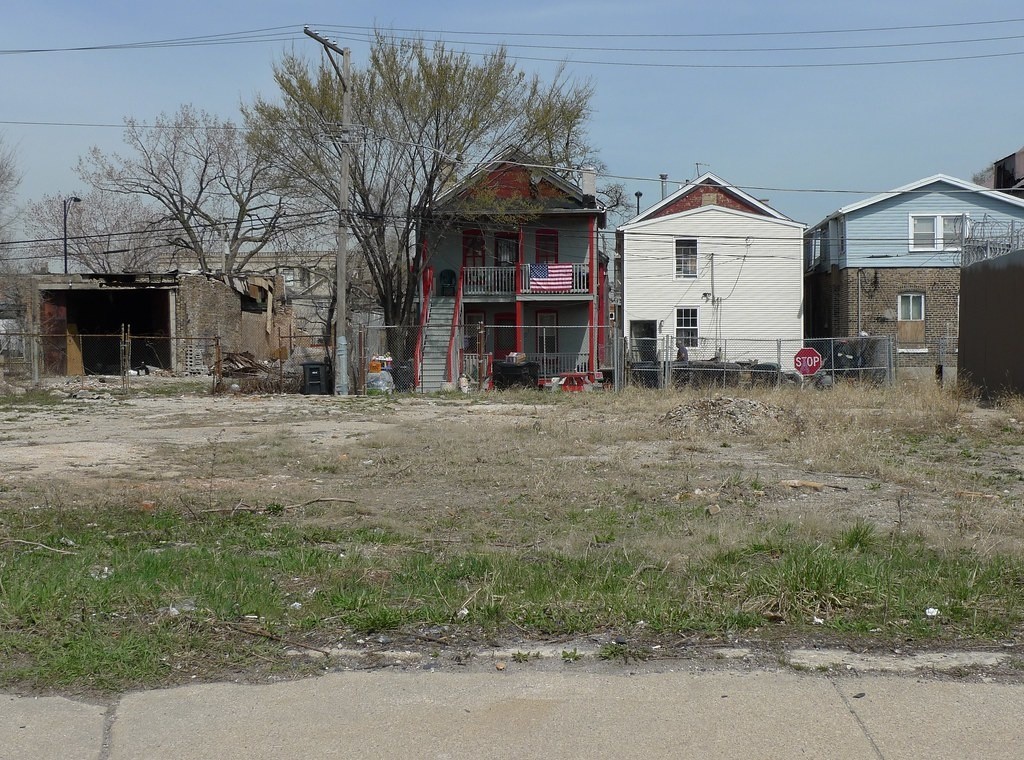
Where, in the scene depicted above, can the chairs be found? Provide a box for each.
[439,269,456,296]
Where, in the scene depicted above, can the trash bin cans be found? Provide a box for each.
[444,283,455,296]
[631,361,778,389]
[492,360,541,389]
[298,362,331,394]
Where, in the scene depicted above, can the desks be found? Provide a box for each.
[560,373,585,391]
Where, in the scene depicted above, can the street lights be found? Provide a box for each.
[635,191,642,216]
[63,197,82,273]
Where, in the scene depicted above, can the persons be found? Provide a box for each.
[676,340,688,361]
[859,324,868,337]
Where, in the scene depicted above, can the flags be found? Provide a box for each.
[530,263,572,290]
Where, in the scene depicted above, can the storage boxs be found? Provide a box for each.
[369,362,381,373]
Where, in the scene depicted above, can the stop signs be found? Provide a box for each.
[795,348,821,376]
